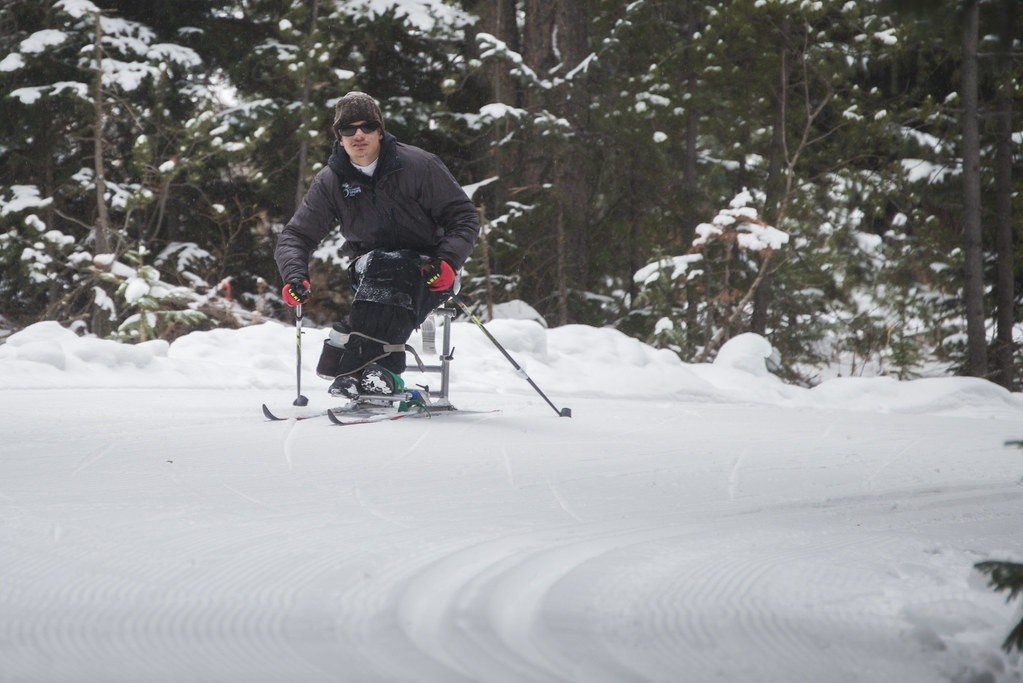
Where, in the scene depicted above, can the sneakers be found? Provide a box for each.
[328,372,363,400]
[358,364,395,397]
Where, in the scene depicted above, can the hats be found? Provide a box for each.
[332,92,385,140]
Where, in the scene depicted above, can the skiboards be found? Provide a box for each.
[261,391,501,426]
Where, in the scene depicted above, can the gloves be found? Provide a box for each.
[282,277,313,308]
[420,255,455,292]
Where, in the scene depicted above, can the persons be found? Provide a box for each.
[275,92,482,400]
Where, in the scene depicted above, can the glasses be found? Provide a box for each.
[338,121,379,136]
